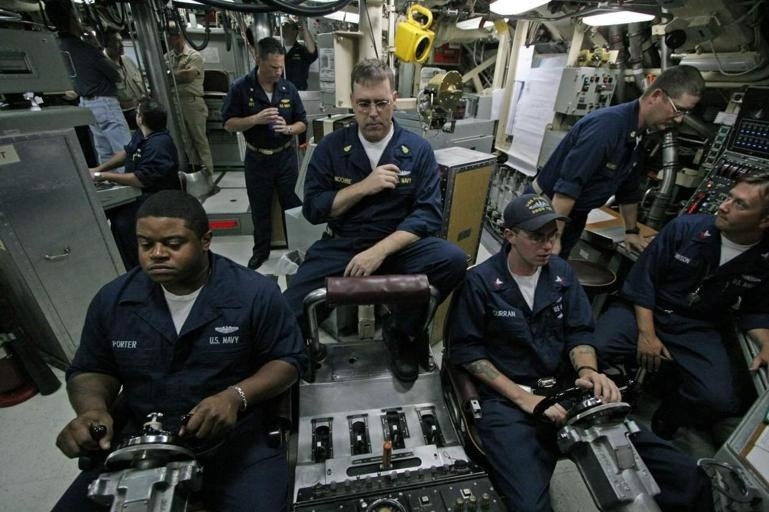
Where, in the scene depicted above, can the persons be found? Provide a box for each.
[162,18,214,176]
[441,193,714,512]
[589,169,768,443]
[222,37,308,267]
[89,97,182,242]
[99,26,148,131]
[51,189,305,511]
[241,16,319,91]
[500,63,705,262]
[44,0,132,173]
[283,59,468,382]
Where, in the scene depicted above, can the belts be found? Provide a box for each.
[241,141,292,157]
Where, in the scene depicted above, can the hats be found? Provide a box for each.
[502,193,572,232]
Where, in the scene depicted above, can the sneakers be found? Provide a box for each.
[379,325,421,383]
[247,256,267,270]
[646,386,688,443]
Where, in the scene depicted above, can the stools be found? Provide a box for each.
[567,259,617,306]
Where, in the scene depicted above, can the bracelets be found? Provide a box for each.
[94,171,102,181]
[625,226,640,235]
[229,384,248,411]
[288,124,292,135]
[575,364,597,375]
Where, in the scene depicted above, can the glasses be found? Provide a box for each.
[353,101,393,111]
[512,229,559,243]
[660,88,691,118]
